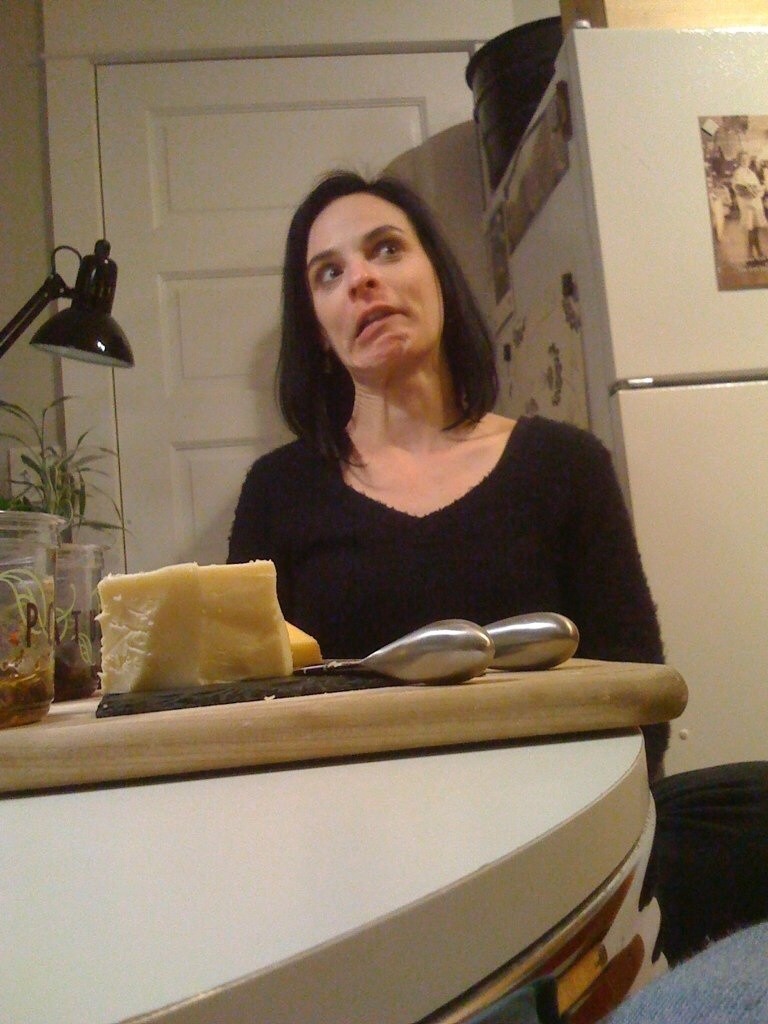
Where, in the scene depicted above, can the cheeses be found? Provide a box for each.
[94,559,322,696]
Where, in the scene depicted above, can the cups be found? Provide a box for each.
[1,509,69,730]
[52,541,110,703]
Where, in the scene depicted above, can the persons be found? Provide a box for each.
[225,171,768,949]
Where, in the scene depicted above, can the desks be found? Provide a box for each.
[0,656,653,1024]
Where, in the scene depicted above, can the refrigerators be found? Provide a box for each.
[469,26,768,785]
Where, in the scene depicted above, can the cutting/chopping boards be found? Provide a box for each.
[0,658,688,800]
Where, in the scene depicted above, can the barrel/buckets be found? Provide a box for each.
[465,15,562,192]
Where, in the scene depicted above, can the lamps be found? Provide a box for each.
[0,236,136,376]
